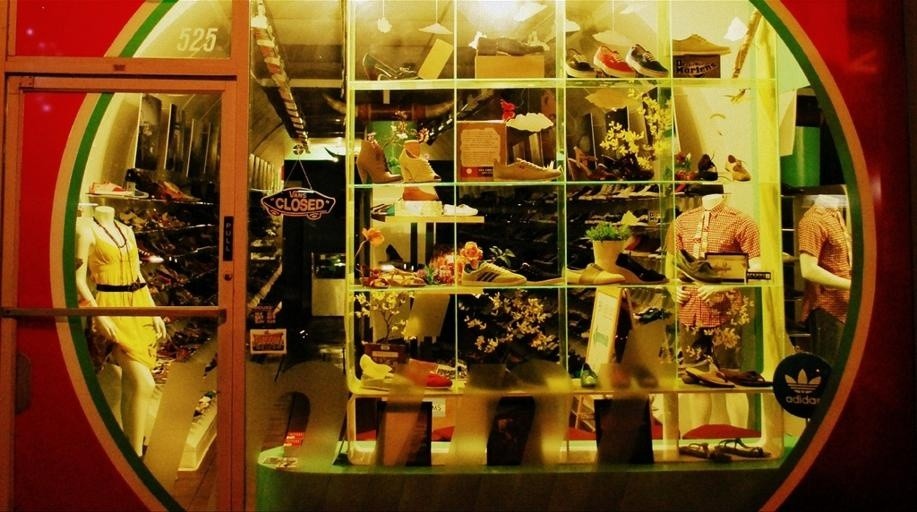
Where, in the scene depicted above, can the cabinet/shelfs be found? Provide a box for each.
[87,193,219,360]
[344,0,785,465]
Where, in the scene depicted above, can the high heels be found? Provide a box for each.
[398,140,443,183]
[616,250,668,284]
[363,52,422,79]
[355,138,403,184]
[567,146,617,182]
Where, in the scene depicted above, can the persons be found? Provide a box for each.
[664,194,761,428]
[74,205,167,460]
[795,193,852,363]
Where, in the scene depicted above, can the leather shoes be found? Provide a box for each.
[493,157,561,182]
[503,349,544,387]
[561,262,625,285]
[580,363,601,387]
[610,361,658,388]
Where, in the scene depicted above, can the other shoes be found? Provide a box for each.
[88,168,218,385]
[247,228,283,366]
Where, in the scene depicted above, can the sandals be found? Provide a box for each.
[477,30,551,56]
[679,355,775,389]
[358,264,427,288]
[679,442,732,461]
[715,436,772,458]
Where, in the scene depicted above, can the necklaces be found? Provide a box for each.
[92,215,133,306]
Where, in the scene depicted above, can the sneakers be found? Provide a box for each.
[629,287,673,322]
[626,44,670,78]
[444,203,479,217]
[787,319,805,331]
[506,257,562,284]
[724,154,752,182]
[782,252,798,263]
[361,366,453,391]
[784,285,803,299]
[592,44,637,78]
[564,47,597,78]
[462,261,527,286]
[671,249,723,283]
[505,154,724,270]
[403,187,439,201]
[672,34,731,58]
